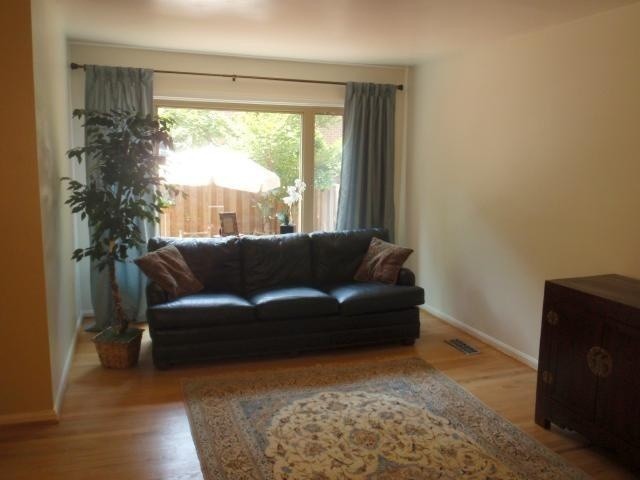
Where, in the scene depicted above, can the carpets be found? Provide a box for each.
[180,356,594,479]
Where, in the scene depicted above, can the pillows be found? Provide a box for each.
[134,244,208,302]
[354,235,416,286]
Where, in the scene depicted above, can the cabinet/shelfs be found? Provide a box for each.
[533,274,640,467]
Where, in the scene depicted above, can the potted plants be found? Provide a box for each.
[61,107,187,371]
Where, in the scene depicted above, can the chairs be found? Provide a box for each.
[217,211,244,237]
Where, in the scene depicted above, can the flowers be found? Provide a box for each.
[276,177,309,224]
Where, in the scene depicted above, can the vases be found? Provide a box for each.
[279,223,294,235]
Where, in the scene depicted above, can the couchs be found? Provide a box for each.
[141,226,427,368]
